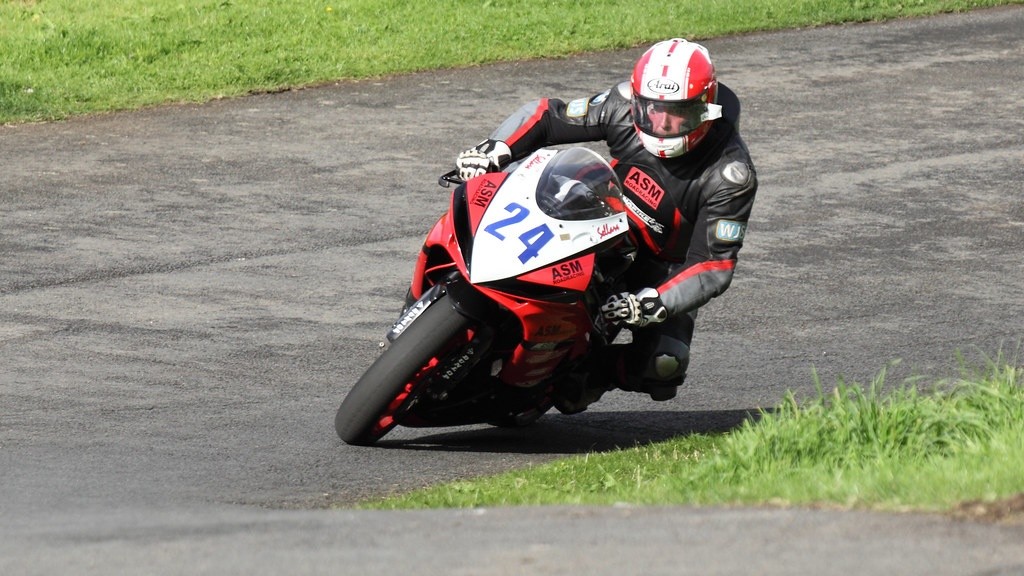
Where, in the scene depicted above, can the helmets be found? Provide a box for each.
[630,37,718,158]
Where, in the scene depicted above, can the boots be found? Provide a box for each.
[554,355,635,413]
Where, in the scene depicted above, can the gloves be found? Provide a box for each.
[600,287,669,327]
[455,139,513,181]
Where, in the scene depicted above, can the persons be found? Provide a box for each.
[456,37,759,415]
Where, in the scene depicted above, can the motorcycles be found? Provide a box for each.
[333,141,630,447]
